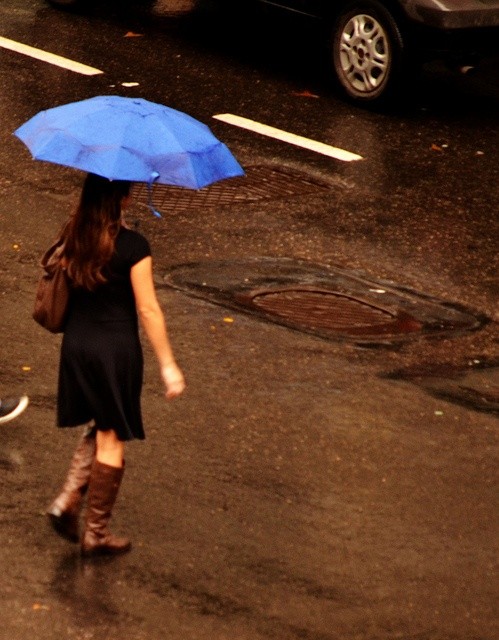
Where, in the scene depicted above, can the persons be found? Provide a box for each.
[42,172,187,557]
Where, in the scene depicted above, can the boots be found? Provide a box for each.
[81,456,132,553]
[46,433,97,543]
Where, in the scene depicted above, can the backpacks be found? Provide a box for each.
[32,219,74,331]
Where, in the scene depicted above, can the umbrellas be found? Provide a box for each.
[12,94,246,194]
